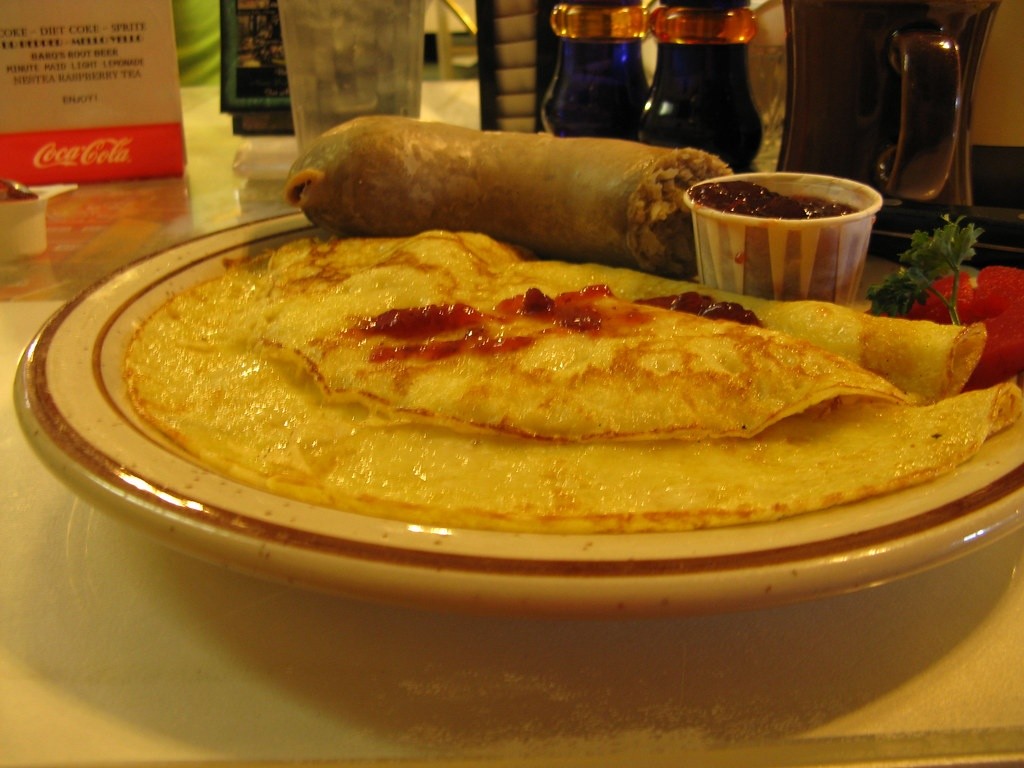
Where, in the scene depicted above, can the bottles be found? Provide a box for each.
[542,0,647,138]
[638,0,760,173]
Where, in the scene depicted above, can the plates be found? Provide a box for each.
[14,213,1024,616]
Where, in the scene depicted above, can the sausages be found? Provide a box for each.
[285,116,738,275]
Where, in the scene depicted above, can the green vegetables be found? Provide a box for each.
[866,216,986,327]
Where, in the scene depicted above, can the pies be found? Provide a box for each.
[124,228,1024,527]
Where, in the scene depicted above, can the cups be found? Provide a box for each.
[747,44,789,172]
[783,0,1001,206]
[278,0,425,159]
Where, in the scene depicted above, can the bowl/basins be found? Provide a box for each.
[683,172,883,307]
